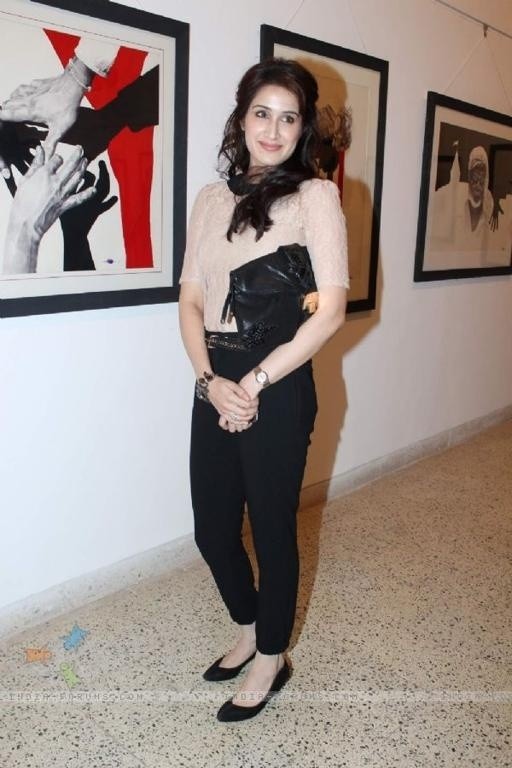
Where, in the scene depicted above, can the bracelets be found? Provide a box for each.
[195,371,214,404]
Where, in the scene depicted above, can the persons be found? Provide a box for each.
[431,145,504,269]
[178,58,350,721]
[1,31,163,275]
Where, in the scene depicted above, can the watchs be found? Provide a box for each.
[253,365,270,390]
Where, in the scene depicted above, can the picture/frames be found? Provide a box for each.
[411,88,511,281]
[0,0,194,321]
[254,21,391,315]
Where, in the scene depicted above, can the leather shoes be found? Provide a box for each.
[201,646,257,680]
[216,657,290,722]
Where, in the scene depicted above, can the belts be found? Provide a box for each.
[205,336,244,352]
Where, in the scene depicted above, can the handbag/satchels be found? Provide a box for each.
[220,244,320,359]
